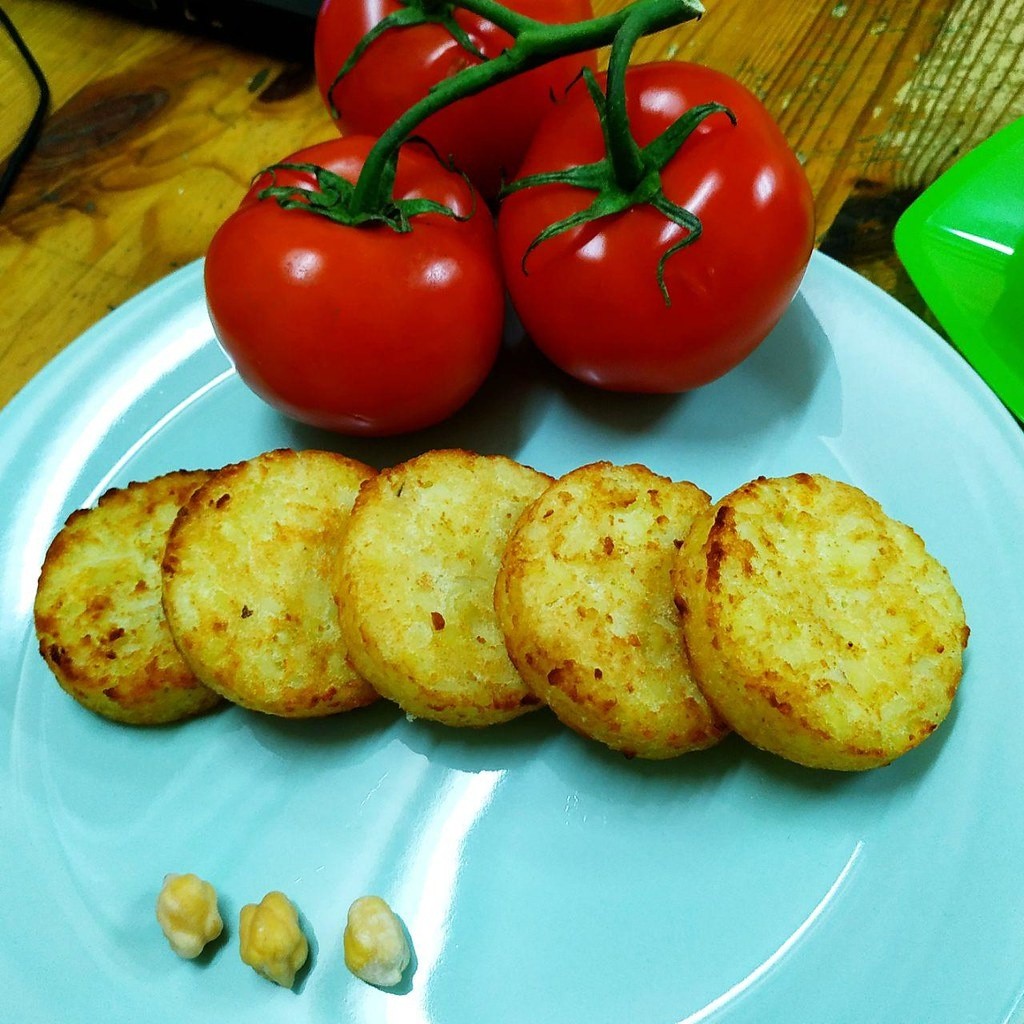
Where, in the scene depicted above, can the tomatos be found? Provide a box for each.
[315,0,599,198]
[496,58,818,396]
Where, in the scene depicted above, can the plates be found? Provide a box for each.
[894,119,1024,424]
[0,248,1024,1024]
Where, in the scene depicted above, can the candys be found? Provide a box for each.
[202,135,508,435]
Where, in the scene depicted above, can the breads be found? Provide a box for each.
[28,448,969,772]
